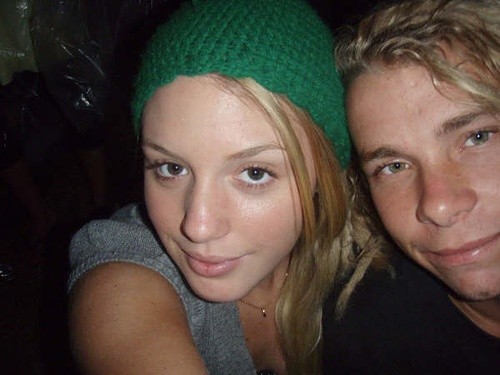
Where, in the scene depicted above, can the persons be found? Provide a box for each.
[318,1,499,374]
[67,1,385,375]
[0,0,120,289]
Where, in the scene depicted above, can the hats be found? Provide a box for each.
[132,0,351,168]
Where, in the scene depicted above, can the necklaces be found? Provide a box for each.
[238,251,294,320]
